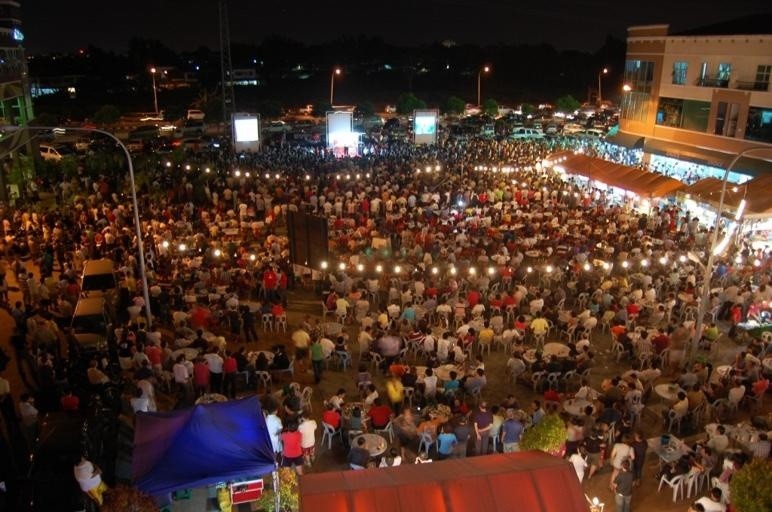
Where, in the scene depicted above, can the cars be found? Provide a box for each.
[265,119,314,133]
[125,124,206,152]
[498,104,606,139]
[385,105,397,114]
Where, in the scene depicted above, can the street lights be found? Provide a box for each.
[599,69,608,100]
[476,67,489,104]
[331,68,343,108]
[52,127,152,324]
[150,70,161,114]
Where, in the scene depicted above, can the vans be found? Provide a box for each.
[70,261,120,353]
[354,116,383,132]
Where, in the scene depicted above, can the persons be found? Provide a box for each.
[1,124,772,510]
